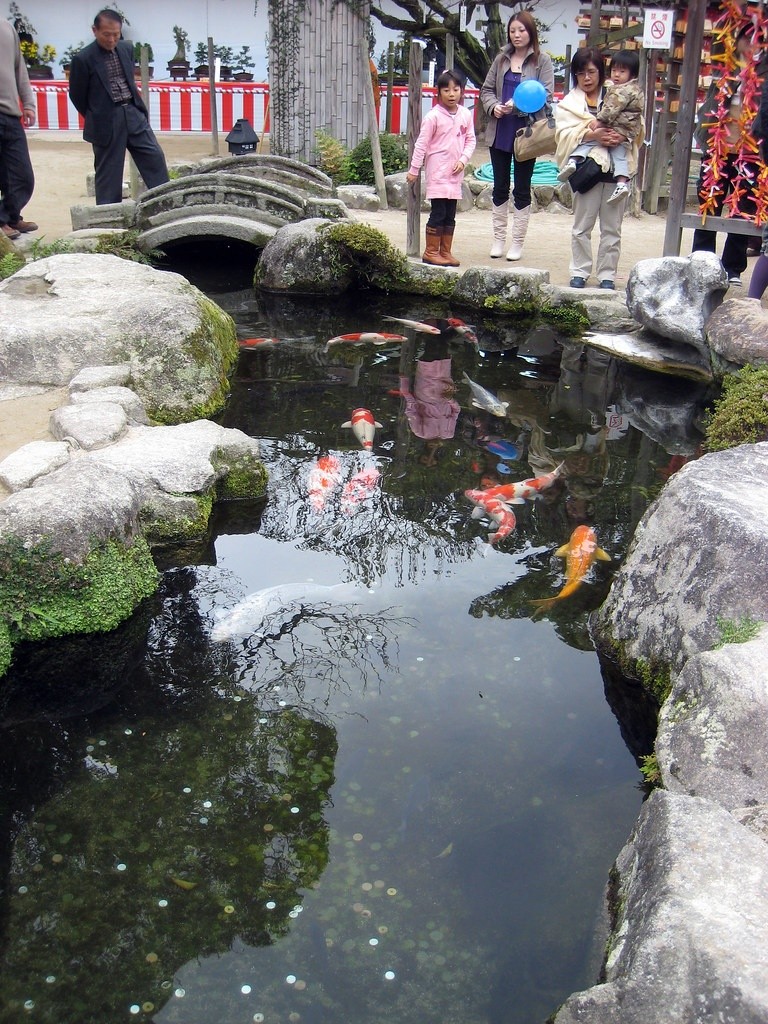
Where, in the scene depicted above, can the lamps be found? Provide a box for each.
[225,118,260,156]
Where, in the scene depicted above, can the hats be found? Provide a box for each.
[733,20,764,42]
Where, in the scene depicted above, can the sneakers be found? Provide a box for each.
[599,280,616,290]
[606,184,630,207]
[556,161,577,183]
[569,276,587,288]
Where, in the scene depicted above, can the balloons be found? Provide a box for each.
[513,79,548,113]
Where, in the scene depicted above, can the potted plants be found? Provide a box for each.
[218,43,233,76]
[132,42,154,77]
[234,46,255,81]
[167,26,192,82]
[59,42,84,79]
[196,42,209,75]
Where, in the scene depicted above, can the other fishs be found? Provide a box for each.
[464,460,564,544]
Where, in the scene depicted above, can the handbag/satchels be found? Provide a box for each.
[568,155,603,195]
[514,101,557,162]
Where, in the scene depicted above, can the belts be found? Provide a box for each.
[112,97,136,107]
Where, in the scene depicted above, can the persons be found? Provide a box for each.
[464,351,525,491]
[556,49,645,205]
[555,45,646,289]
[69,9,170,205]
[405,69,477,267]
[480,11,555,261]
[526,343,610,524]
[0,19,39,239]
[691,0,768,299]
[400,339,462,469]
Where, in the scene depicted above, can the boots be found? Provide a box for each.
[506,202,532,261]
[422,224,461,267]
[488,198,510,258]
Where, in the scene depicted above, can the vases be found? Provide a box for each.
[27,67,54,81]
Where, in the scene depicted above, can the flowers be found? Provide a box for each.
[22,42,55,65]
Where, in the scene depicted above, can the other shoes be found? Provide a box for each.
[746,248,761,257]
[1,219,39,238]
[729,277,743,287]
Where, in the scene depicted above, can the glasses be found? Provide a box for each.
[575,68,599,80]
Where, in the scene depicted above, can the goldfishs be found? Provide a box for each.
[322,332,408,354]
[382,314,441,334]
[306,407,383,519]
[460,371,509,417]
[526,524,612,623]
[239,338,280,348]
[211,580,356,645]
[444,317,480,352]
